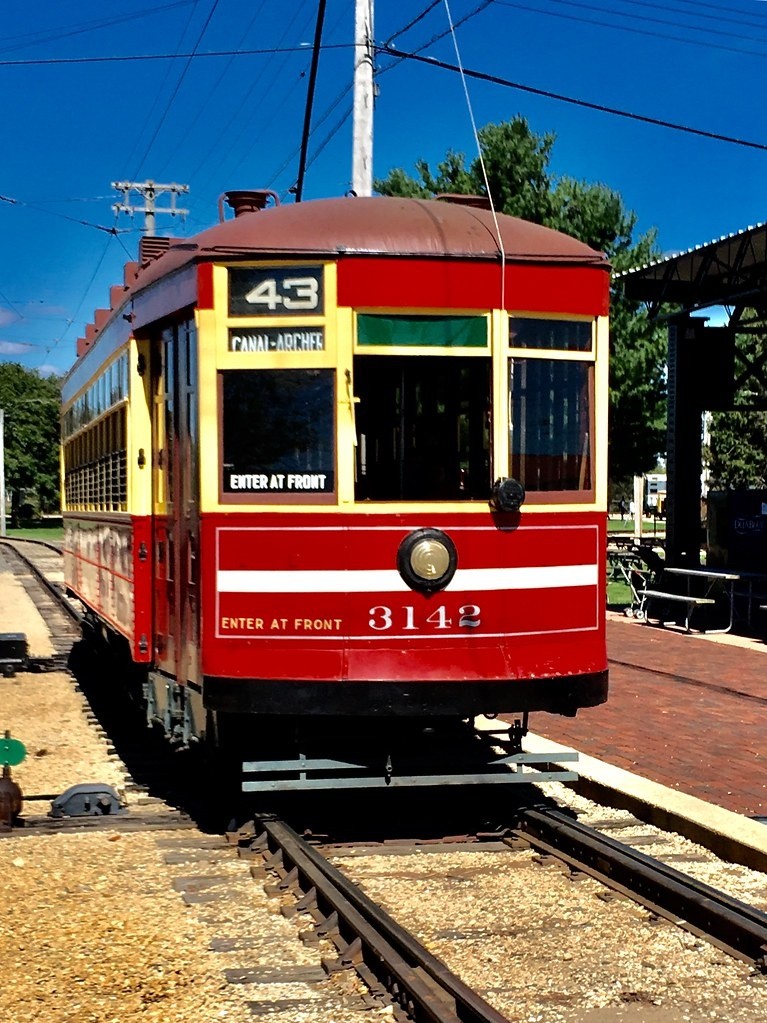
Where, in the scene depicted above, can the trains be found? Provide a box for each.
[59,196,615,821]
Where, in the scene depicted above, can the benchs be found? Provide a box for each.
[607,532,740,635]
